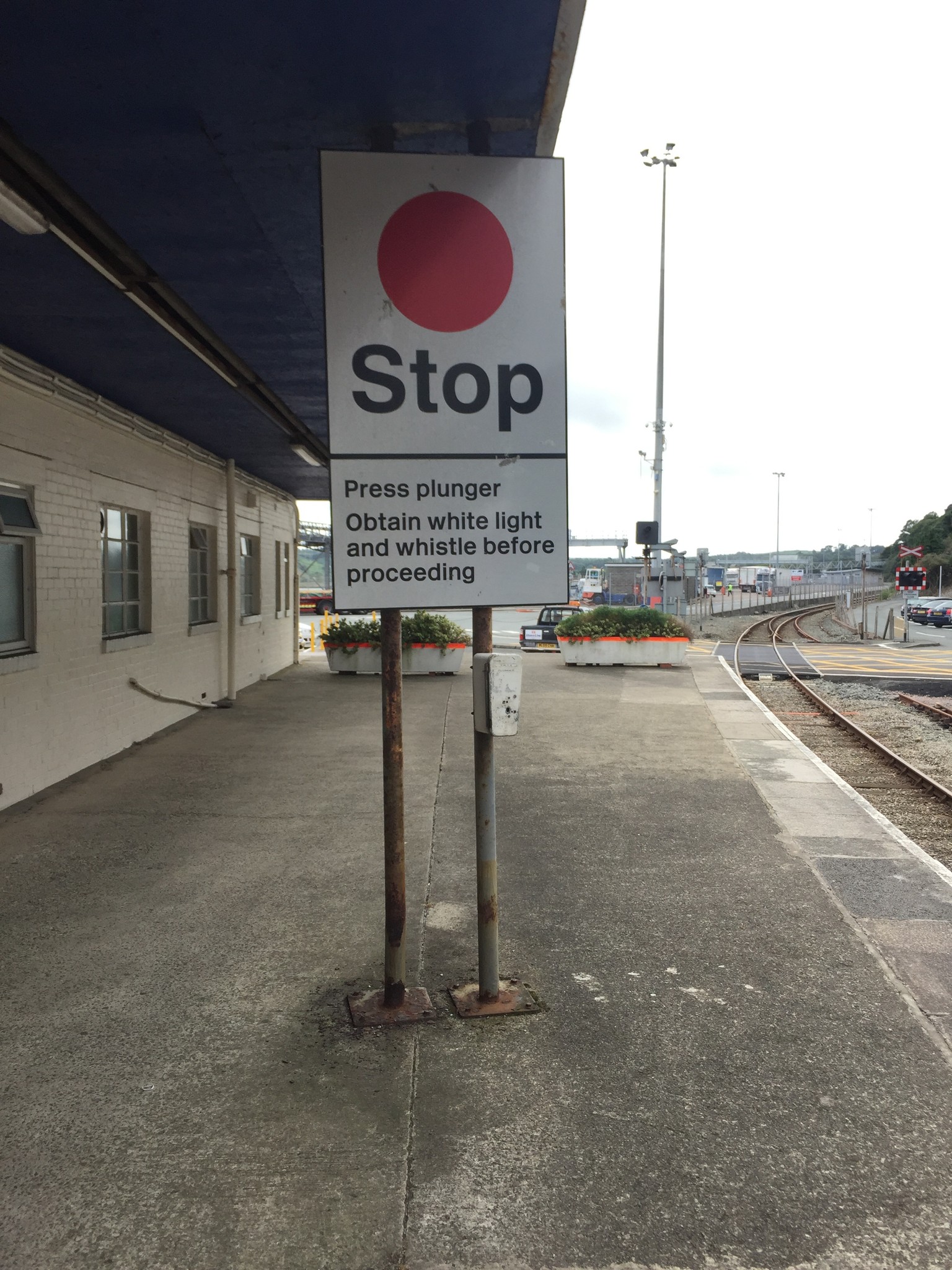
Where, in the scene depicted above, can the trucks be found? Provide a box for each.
[698,563,790,595]
[581,563,650,605]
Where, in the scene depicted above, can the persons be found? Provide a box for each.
[727,582,732,597]
[703,586,707,598]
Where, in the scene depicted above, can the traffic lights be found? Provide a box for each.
[900,570,925,586]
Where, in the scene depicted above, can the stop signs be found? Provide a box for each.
[323,152,566,614]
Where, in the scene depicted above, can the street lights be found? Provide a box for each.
[868,507,875,567]
[771,473,785,567]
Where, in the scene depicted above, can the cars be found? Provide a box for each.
[902,596,938,621]
[697,585,717,596]
[928,601,952,628]
[911,597,952,627]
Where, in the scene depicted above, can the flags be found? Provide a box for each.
[833,535,839,545]
[868,508,872,511]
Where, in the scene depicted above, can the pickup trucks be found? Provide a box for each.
[520,607,594,653]
